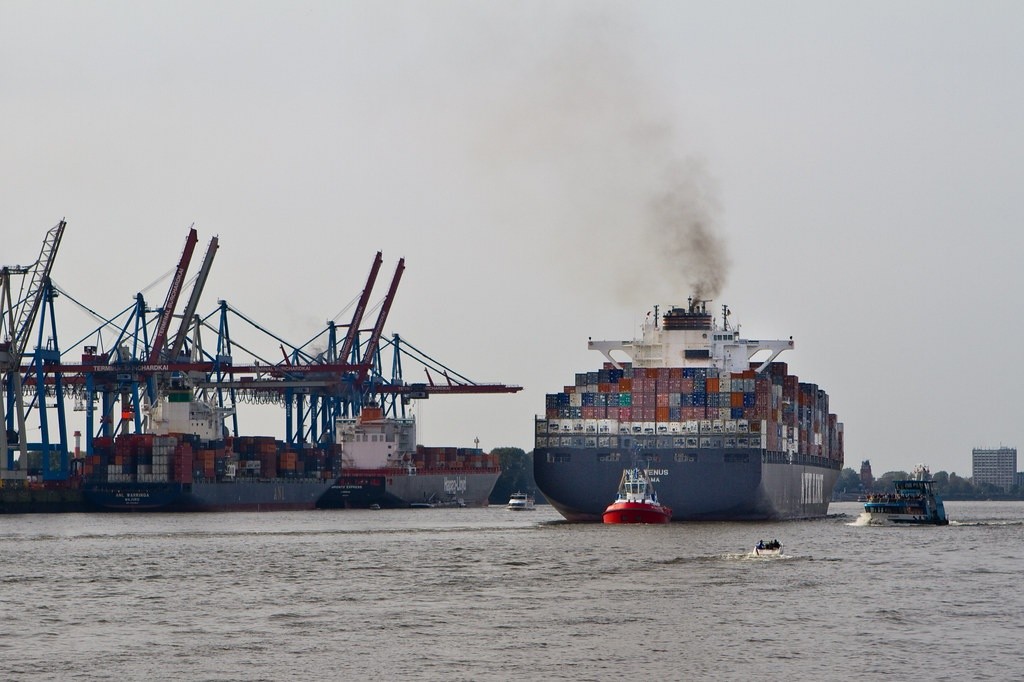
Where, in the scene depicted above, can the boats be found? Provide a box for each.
[409,489,466,508]
[601,460,674,525]
[752,539,784,556]
[864,464,950,525]
[506,490,536,511]
[370,503,381,511]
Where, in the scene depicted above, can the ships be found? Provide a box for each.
[531,296,846,523]
[0,376,505,513]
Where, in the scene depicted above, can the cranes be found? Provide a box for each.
[0,218,522,482]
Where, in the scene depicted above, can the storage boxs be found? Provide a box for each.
[412,447,500,469]
[85,431,342,483]
[542,363,844,462]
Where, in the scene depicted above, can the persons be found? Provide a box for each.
[864,491,924,502]
[774,539,779,548]
[769,540,773,549]
[757,539,764,549]
